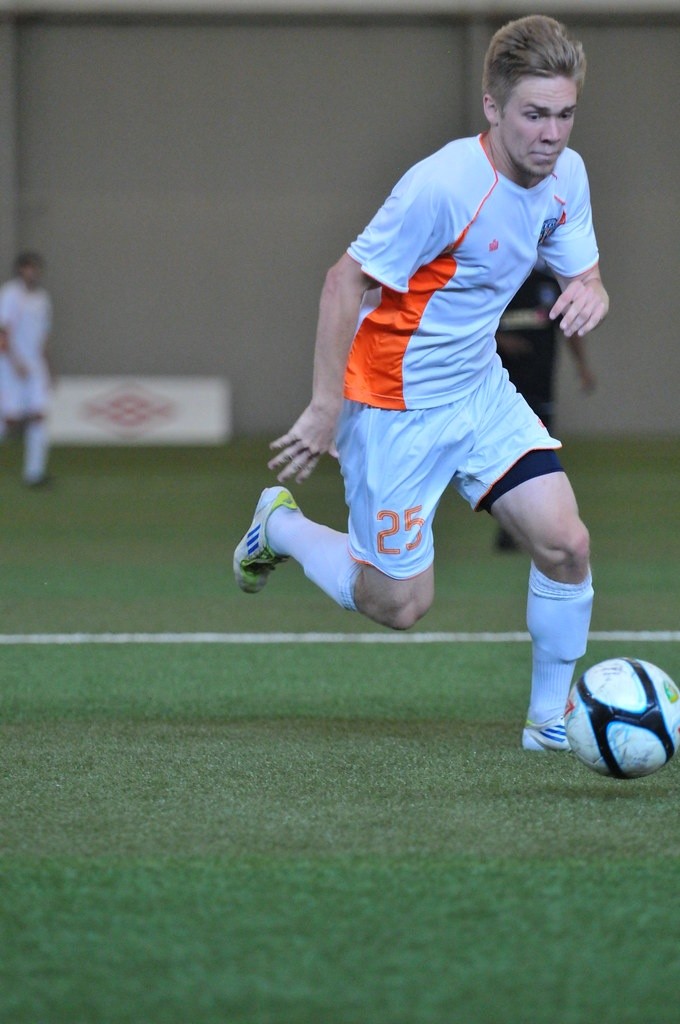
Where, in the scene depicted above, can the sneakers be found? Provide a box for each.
[522,715,573,752]
[233,485,298,594]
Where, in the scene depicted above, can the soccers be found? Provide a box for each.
[565,657,680,780]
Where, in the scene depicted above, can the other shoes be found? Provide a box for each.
[498,528,519,550]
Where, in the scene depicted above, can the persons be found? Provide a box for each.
[0,253,57,488]
[499,263,595,551]
[233,13,612,751]
